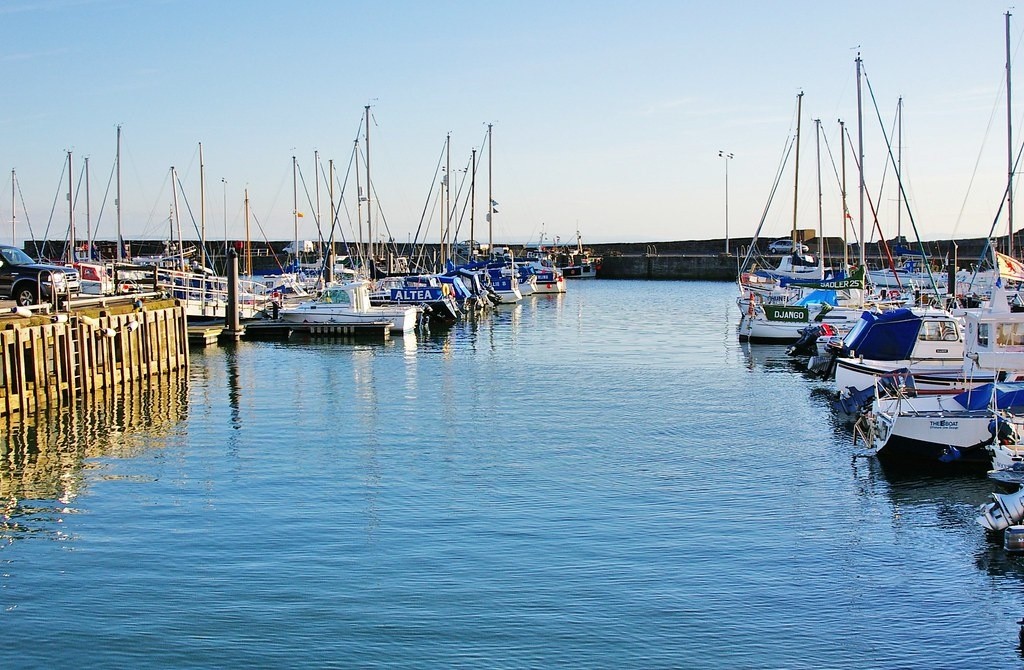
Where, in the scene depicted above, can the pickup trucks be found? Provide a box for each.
[453,240,489,254]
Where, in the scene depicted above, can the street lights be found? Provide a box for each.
[719,150,734,254]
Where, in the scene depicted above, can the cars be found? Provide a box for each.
[768,240,809,255]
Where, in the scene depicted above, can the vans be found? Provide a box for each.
[282,240,314,255]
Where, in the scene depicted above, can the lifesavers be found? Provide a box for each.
[886,290,900,299]
[443,284,449,296]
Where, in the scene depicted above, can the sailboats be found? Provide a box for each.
[10,104,596,336]
[735,7,1023,552]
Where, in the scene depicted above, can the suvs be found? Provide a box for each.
[0,245,82,311]
